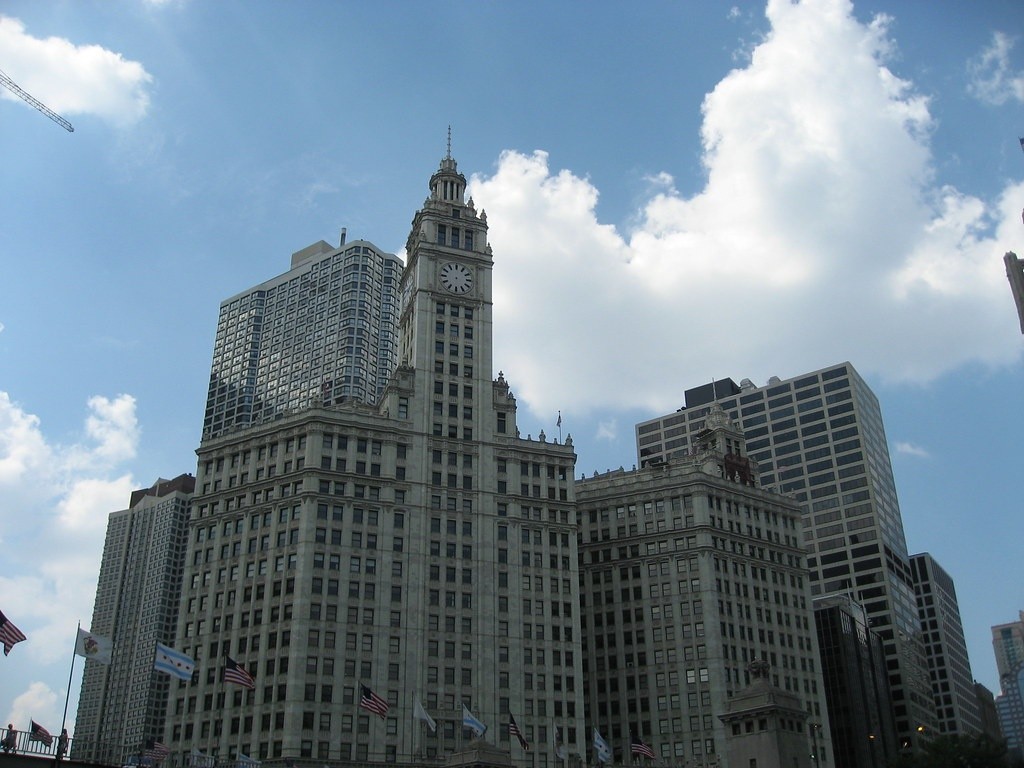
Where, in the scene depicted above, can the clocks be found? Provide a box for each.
[438,262,475,294]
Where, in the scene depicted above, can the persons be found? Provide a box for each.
[6,724,16,752]
[59,729,67,759]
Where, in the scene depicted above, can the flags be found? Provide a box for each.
[413,693,436,732]
[76,628,112,665]
[557,415,561,426]
[143,741,261,768]
[462,704,486,736]
[593,729,610,762]
[1,613,26,656]
[154,641,196,681]
[510,712,528,750]
[30,721,53,747]
[360,684,389,720]
[224,655,256,689]
[631,734,657,759]
[553,723,565,759]
